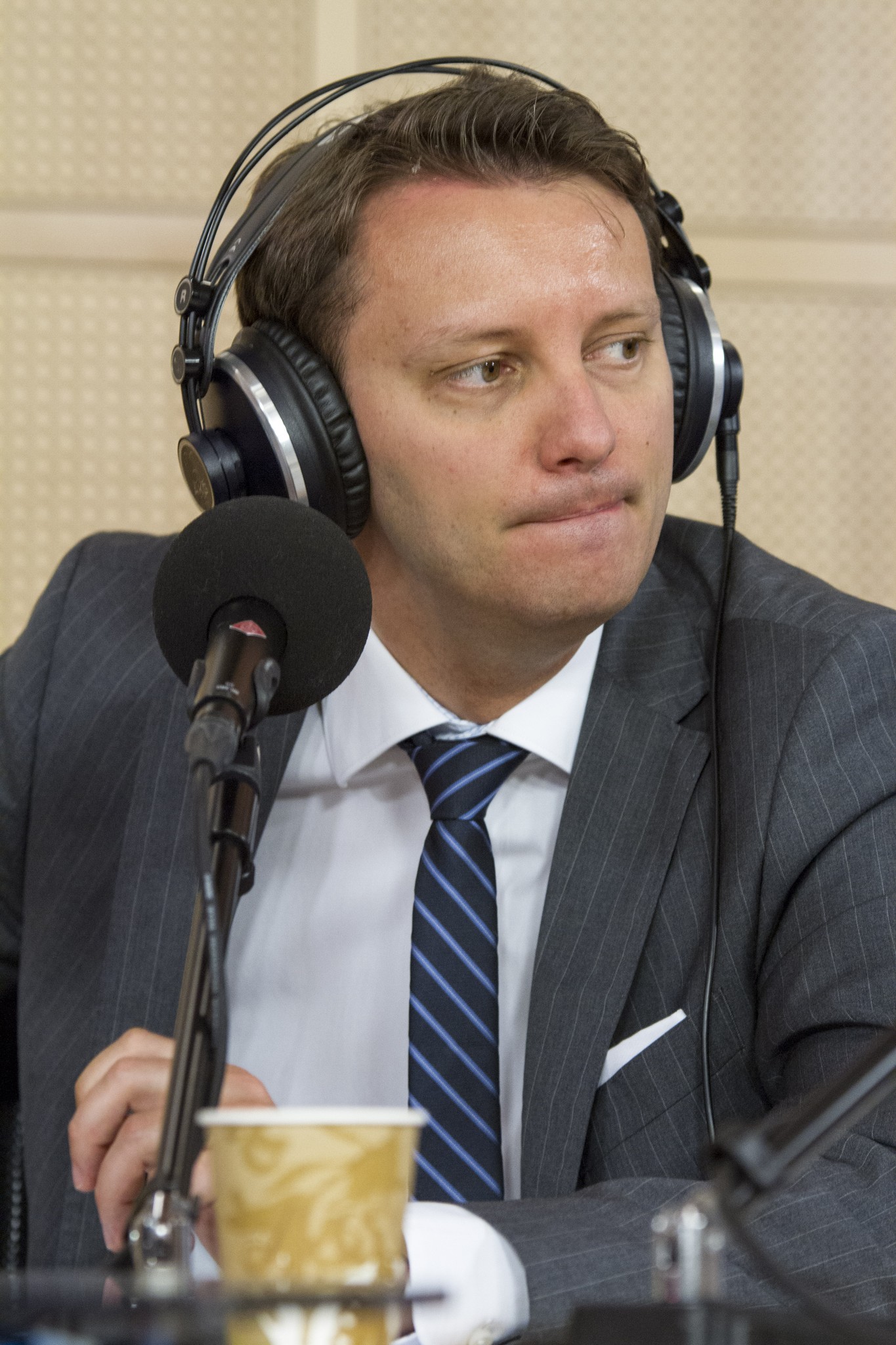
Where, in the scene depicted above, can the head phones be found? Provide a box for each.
[170,52,743,542]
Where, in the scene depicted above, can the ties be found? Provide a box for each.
[396,727,528,1200]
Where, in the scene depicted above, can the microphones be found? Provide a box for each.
[152,501,372,769]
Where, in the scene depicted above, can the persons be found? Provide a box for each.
[1,63,894,1343]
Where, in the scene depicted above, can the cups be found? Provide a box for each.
[196,1094,432,1344]
